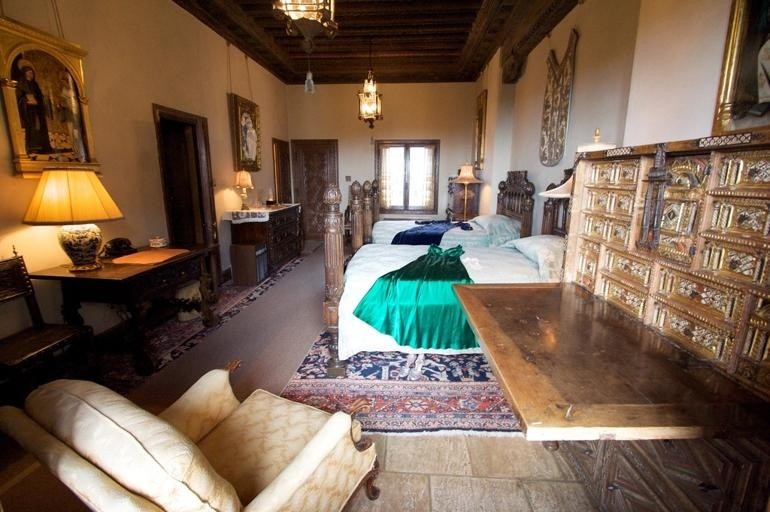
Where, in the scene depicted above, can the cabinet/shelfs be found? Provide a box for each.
[229,203,302,271]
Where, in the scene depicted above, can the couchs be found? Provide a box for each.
[0,357,380,512]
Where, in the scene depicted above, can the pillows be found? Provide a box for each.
[473,213,520,232]
[505,231,564,272]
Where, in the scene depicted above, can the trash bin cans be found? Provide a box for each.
[174,280,203,321]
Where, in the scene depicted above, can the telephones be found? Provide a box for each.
[104,238,138,256]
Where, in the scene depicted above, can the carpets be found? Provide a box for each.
[280,326,520,435]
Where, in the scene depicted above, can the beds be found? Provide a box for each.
[363,169,534,243]
[321,170,574,345]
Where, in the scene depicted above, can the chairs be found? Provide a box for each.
[0,244,93,378]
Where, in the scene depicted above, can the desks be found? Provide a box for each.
[32,247,214,366]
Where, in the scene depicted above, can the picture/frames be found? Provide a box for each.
[475,89,486,178]
[3,23,103,170]
[227,94,262,171]
[709,0,765,131]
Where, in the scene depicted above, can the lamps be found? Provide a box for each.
[22,168,123,275]
[357,38,383,129]
[272,0,339,53]
[303,54,318,96]
[235,170,255,210]
[538,129,617,201]
[453,159,483,218]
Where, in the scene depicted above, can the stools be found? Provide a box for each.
[229,242,270,285]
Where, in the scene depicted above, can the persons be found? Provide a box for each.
[245,117,256,160]
[57,78,79,156]
[14,65,52,155]
